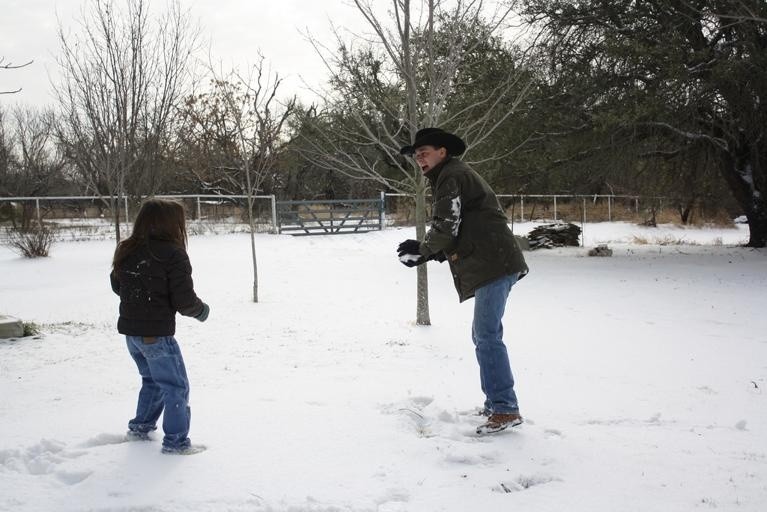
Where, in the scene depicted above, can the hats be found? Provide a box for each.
[400,128,466,157]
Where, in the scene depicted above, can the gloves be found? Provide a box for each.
[398,239,433,267]
[194,304,210,322]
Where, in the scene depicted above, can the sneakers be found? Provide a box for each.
[123,431,153,442]
[472,410,524,435]
[161,446,207,454]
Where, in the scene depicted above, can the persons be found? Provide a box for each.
[108,198,210,455]
[396,128,530,435]
